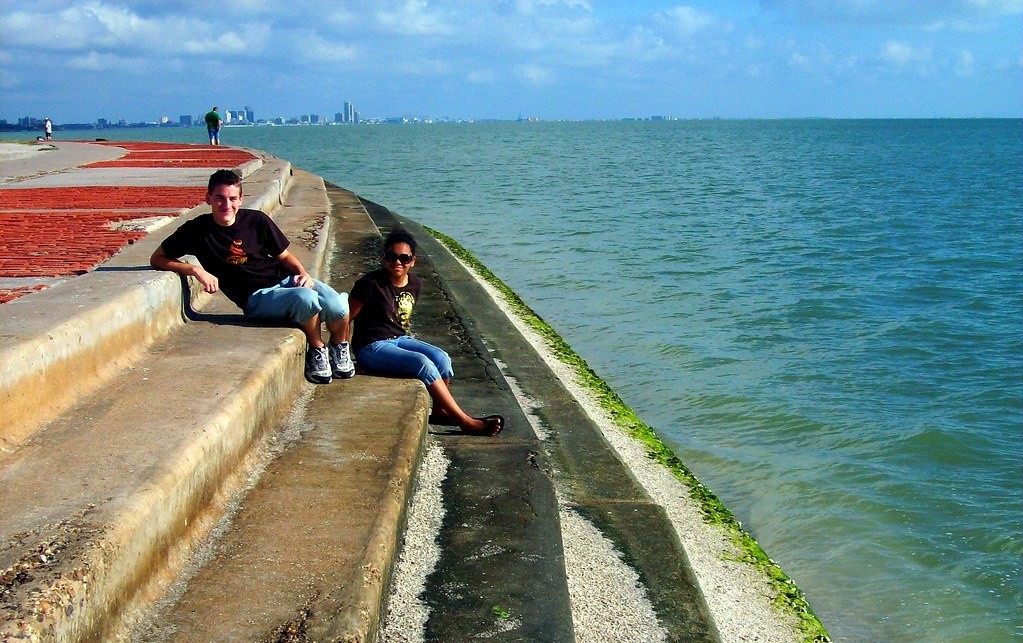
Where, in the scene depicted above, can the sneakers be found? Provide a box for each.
[328,340,355,379]
[307,342,332,384]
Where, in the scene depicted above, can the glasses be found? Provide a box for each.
[385,251,413,264]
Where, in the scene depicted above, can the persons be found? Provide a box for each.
[348,228,506,436]
[45,117,52,140]
[149,169,357,383]
[205,107,222,145]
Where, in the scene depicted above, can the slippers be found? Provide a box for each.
[429,414,460,425]
[472,414,505,437]
